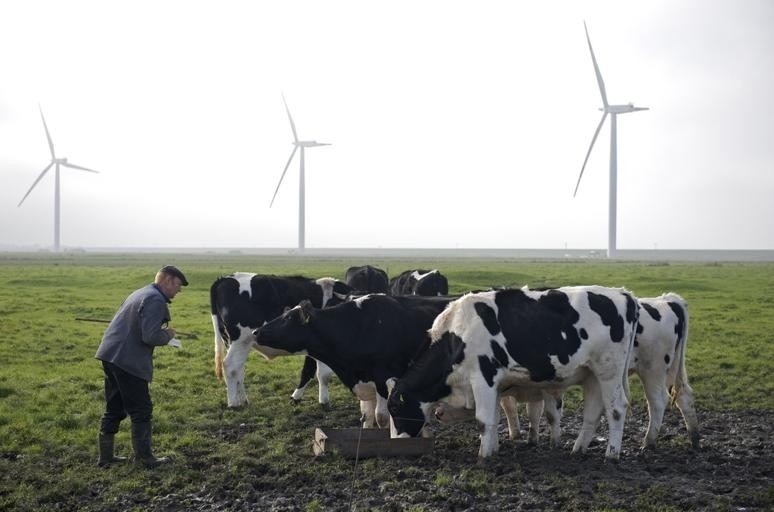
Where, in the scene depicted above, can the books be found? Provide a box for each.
[166,338,181,348]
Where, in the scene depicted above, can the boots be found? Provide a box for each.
[97,432,128,466]
[131,421,165,468]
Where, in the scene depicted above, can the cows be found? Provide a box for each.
[206,262,702,463]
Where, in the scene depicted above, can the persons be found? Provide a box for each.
[93,263,192,468]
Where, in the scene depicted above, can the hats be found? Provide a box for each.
[159,265,189,286]
